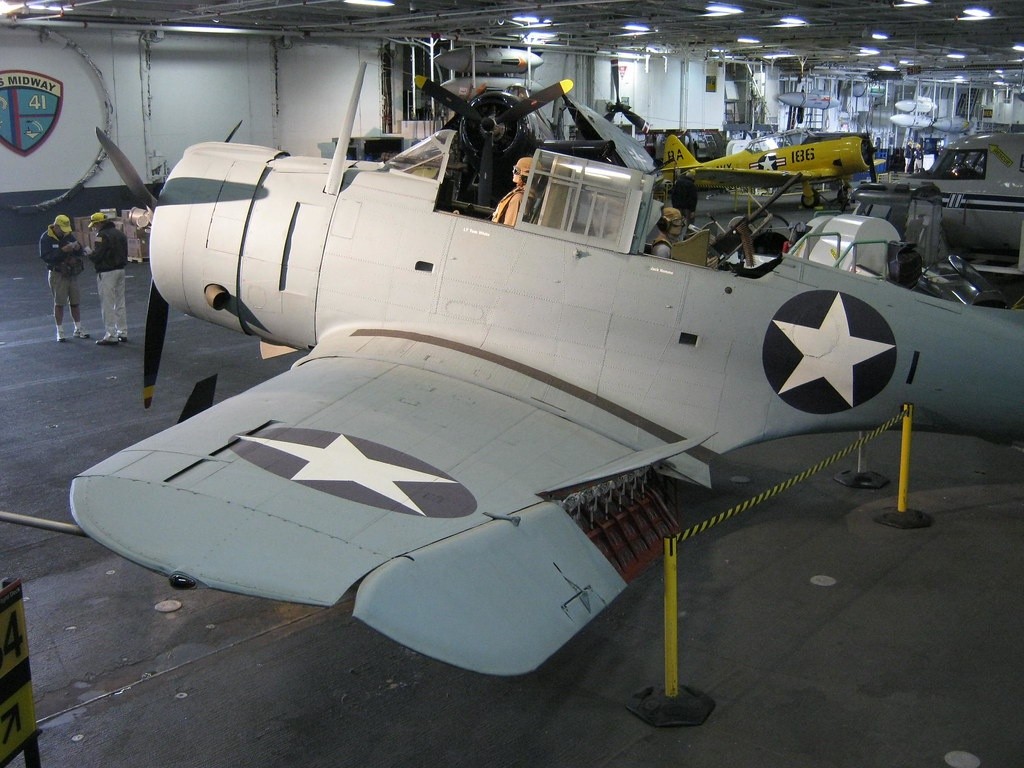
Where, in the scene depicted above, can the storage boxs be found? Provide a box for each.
[71,207,154,259]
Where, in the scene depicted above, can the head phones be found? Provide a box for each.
[657,209,668,234]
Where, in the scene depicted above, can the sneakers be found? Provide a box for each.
[73,329,90,339]
[57,330,68,342]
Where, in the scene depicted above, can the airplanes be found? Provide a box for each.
[69,63,1023,677]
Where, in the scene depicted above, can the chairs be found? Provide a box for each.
[888,240,923,288]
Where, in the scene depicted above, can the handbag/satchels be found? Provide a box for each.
[59,257,85,277]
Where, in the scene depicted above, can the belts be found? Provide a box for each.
[96,265,124,273]
[47,265,60,272]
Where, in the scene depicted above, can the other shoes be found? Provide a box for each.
[118,336,127,342]
[96,339,118,346]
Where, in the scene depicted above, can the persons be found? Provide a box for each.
[903,142,923,172]
[651,208,718,269]
[39,215,91,342]
[83,213,128,344]
[671,169,697,221]
[491,157,541,225]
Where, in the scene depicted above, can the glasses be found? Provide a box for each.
[672,218,687,228]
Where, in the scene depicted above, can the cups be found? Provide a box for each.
[84,246,92,256]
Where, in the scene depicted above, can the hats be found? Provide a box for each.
[513,157,542,177]
[54,215,72,232]
[88,212,107,227]
[662,207,681,220]
